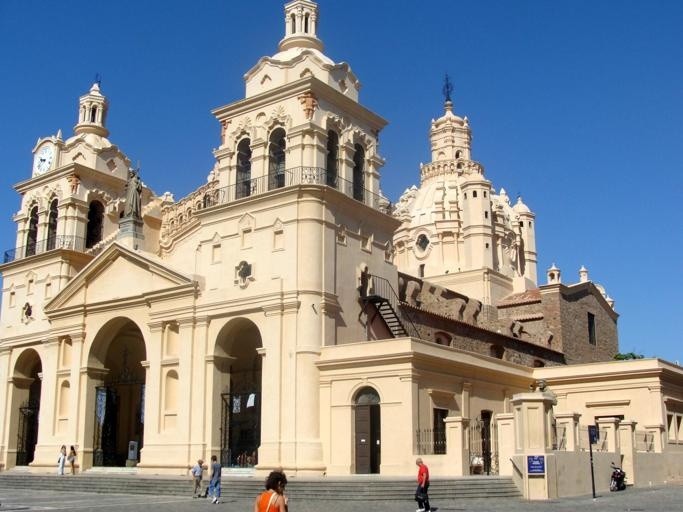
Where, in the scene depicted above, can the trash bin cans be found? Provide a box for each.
[128,441,139,460]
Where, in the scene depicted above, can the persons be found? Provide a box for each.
[58,445,66,475]
[67,445,77,475]
[415,457,431,512]
[482,450,491,475]
[208,455,222,504]
[191,459,204,498]
[237,446,258,467]
[470,453,482,464]
[254,471,288,512]
[124,170,143,220]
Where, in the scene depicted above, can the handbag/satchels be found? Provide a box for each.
[416,488,426,502]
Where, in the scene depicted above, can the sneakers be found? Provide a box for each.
[415,507,431,512]
[211,497,219,504]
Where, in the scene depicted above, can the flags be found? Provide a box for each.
[96,387,108,425]
[246,393,256,408]
[231,394,241,414]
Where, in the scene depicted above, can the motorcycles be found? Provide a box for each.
[609,462,626,491]
